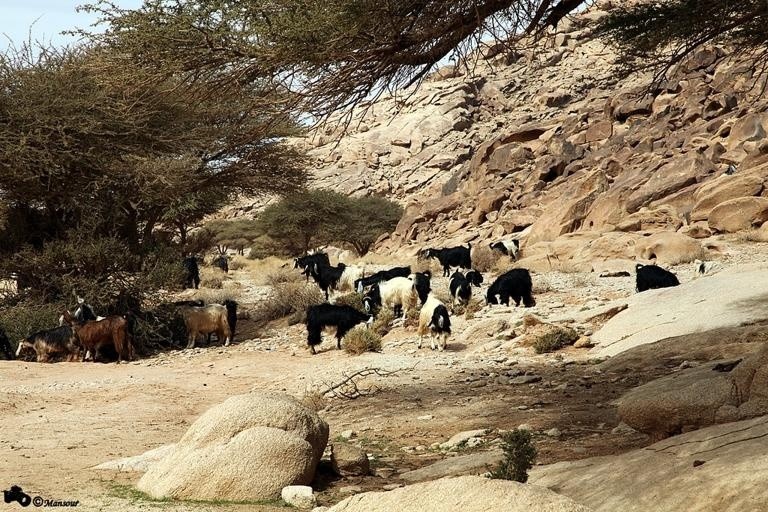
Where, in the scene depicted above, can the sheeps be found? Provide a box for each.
[175,303,231,352]
[201,298,239,343]
[635,264,680,292]
[695,260,723,276]
[292,242,483,354]
[484,268,536,307]
[15,303,135,364]
[218,256,228,272]
[182,257,202,290]
[489,239,519,263]
[157,299,204,325]
